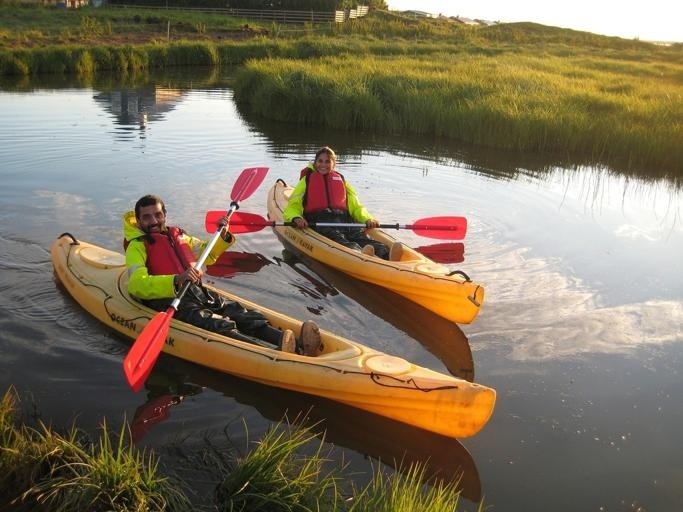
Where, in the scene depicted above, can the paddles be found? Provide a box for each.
[122,166,270,393]
[205,211,467,240]
[207,243,464,278]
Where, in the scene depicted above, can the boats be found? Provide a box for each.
[267,180,483,325]
[51,233,497,440]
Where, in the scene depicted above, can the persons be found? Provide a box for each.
[121,195,321,356]
[283,146,403,261]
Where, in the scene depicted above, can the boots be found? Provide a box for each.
[360,238,402,261]
[337,238,374,256]
[263,320,320,357]
[230,329,295,353]
